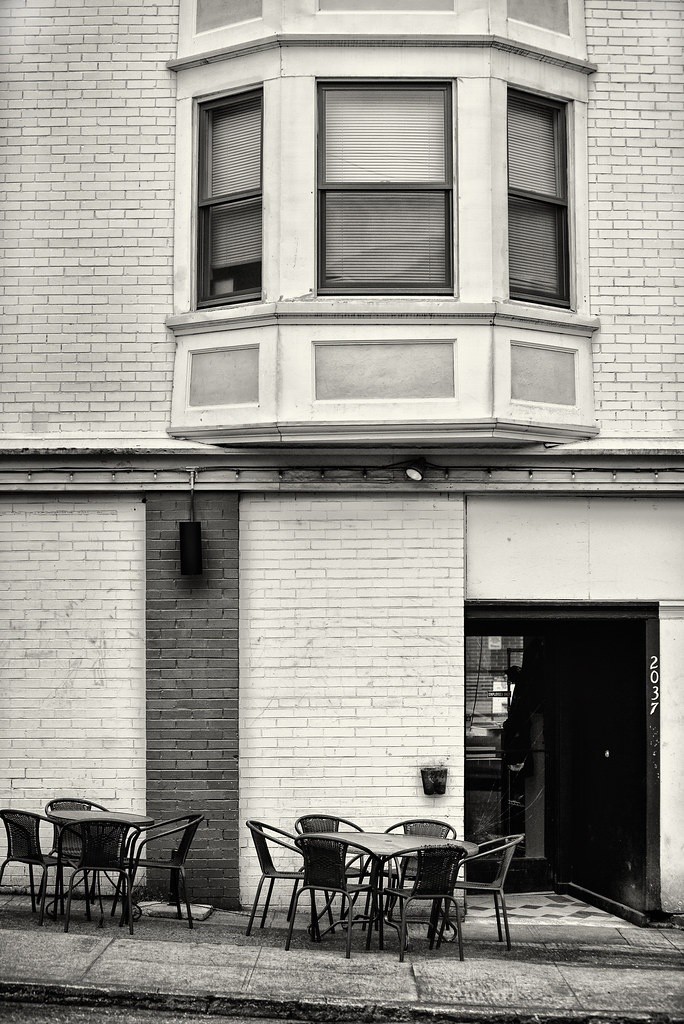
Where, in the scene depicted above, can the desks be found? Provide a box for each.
[47,810,155,929]
[298,832,479,944]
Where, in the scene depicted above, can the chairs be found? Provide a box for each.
[246,813,524,962]
[0,798,205,935]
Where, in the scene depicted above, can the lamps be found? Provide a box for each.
[406,457,426,481]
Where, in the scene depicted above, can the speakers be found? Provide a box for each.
[179,521,202,575]
